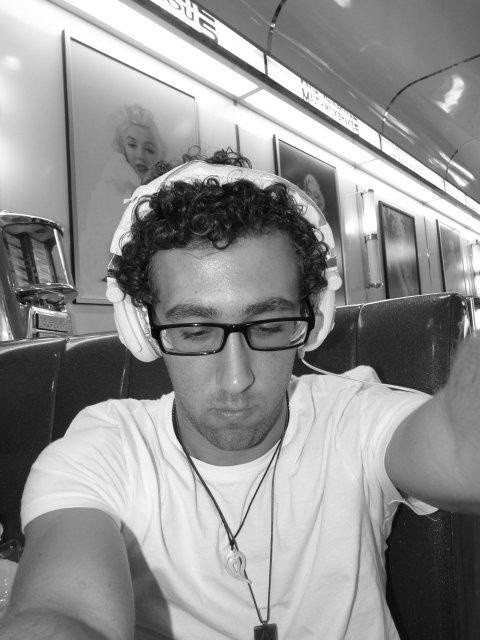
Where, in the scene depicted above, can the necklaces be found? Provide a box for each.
[171,405,291,584]
[169,390,291,639]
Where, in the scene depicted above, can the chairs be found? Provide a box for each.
[0,294,479,638]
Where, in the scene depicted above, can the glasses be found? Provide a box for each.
[147,296,316,356]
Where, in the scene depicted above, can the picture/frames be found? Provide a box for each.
[62,33,206,301]
[435,217,469,296]
[376,198,424,294]
[270,130,352,306]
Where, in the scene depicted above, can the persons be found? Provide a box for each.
[303,174,325,215]
[83,104,164,279]
[387,211,414,298]
[0,143,480,640]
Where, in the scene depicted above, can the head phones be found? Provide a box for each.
[105,160,342,363]
